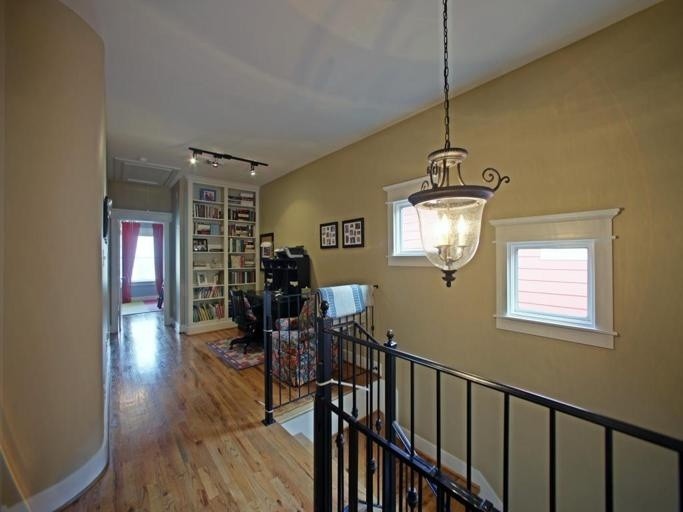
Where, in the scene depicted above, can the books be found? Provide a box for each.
[192,183,256,324]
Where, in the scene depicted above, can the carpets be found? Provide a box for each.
[206,337,265,371]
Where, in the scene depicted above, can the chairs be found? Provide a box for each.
[230,290,342,387]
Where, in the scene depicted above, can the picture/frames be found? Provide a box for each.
[320,217,365,249]
[104,196,111,244]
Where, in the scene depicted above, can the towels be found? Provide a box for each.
[315,285,373,320]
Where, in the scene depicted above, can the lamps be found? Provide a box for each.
[187,147,269,175]
[407,0,508,288]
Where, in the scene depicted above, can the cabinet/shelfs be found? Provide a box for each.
[184,175,309,336]
